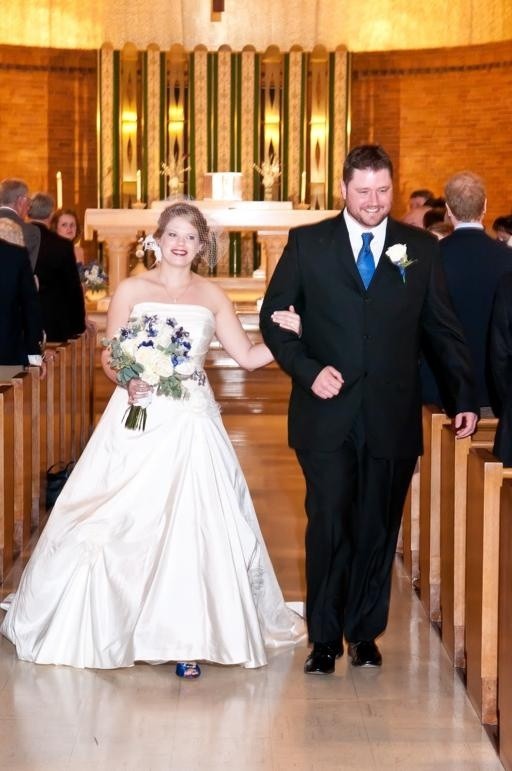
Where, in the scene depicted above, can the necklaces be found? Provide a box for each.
[156,278,192,303]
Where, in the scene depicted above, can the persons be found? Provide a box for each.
[50,210,97,330]
[260,145,481,674]
[406,174,511,474]
[0,180,308,679]
[1,217,46,380]
[1,180,56,358]
[28,190,90,342]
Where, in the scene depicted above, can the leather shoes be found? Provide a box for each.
[346,640,382,669]
[303,637,345,678]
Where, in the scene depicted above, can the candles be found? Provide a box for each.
[301,172,306,203]
[57,171,62,209]
[136,170,142,202]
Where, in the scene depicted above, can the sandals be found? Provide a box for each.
[175,660,201,681]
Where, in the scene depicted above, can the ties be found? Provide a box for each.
[355,233,376,293]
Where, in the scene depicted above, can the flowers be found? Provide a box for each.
[385,243,418,287]
[76,261,109,294]
[101,314,196,431]
